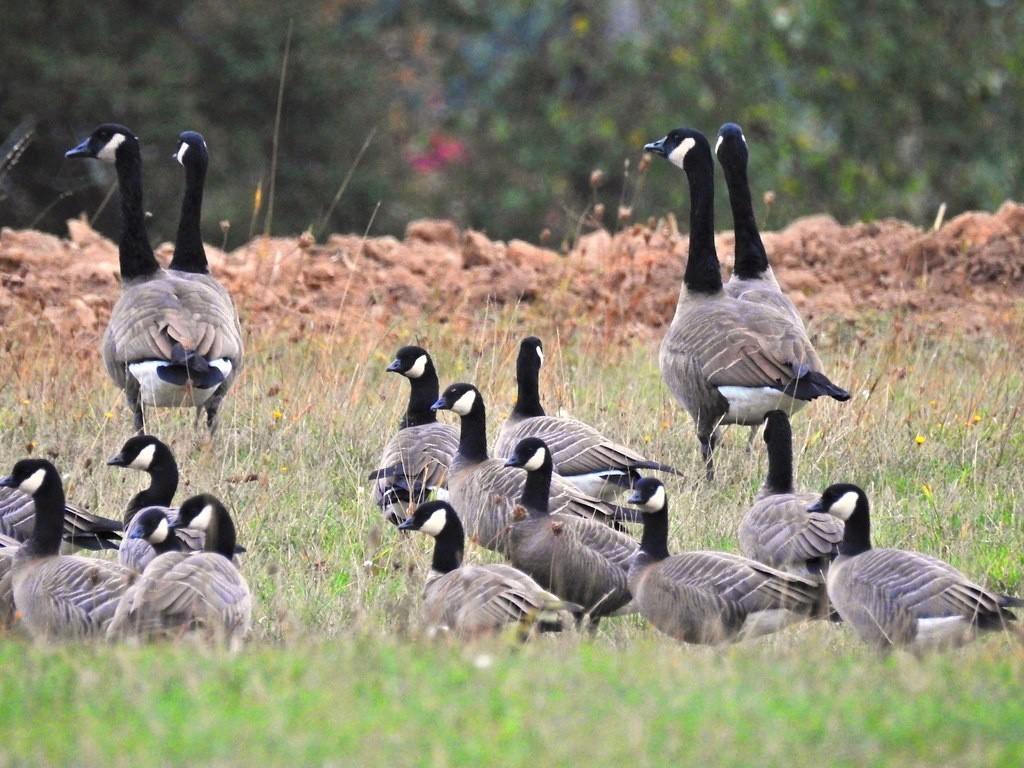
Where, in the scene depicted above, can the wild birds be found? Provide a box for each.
[60,122,244,435]
[0,435,251,646]
[367,336,1024,656]
[642,124,851,480]
